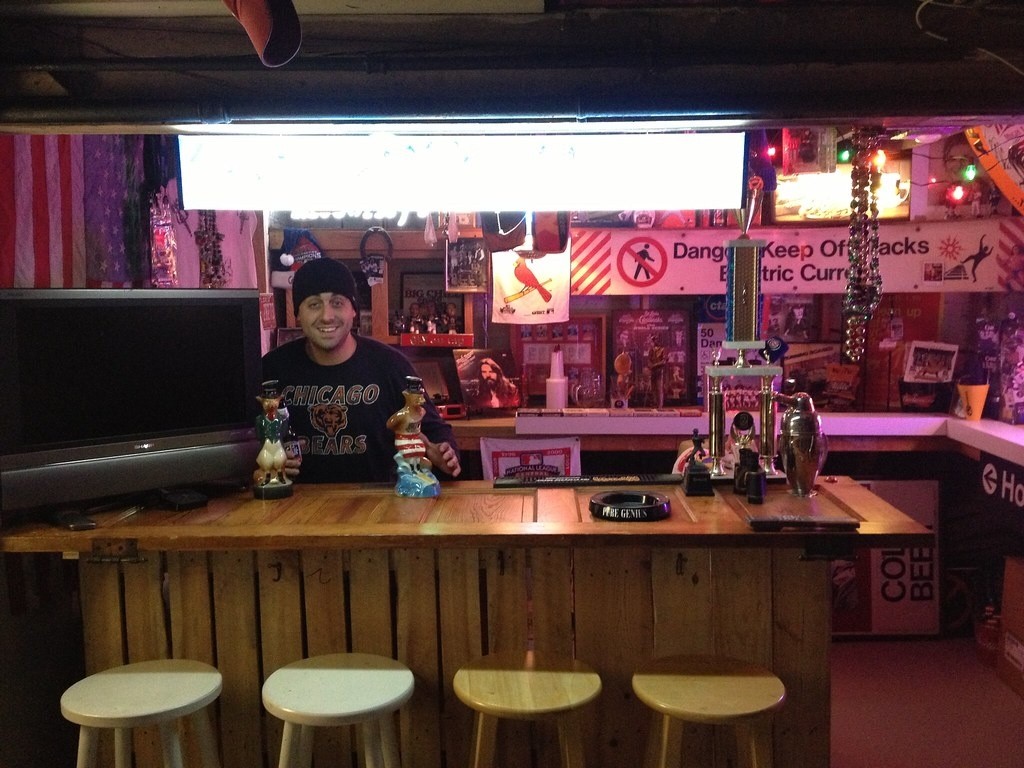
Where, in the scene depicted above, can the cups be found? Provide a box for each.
[957,384,990,422]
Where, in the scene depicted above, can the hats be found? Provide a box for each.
[294,257,359,318]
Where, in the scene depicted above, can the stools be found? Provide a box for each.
[262,653,415,768]
[453,650,603,768]
[60,658,223,768]
[632,652,788,768]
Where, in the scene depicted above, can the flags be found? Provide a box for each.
[0,131,184,288]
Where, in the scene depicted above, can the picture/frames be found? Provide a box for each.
[445,236,491,294]
[779,339,843,408]
[400,272,465,334]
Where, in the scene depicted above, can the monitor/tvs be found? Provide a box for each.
[0,287,264,530]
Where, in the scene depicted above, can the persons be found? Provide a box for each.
[945,182,961,220]
[968,179,985,218]
[647,335,669,411]
[230,255,462,484]
[614,353,632,395]
[407,301,461,327]
[988,180,1000,216]
[451,242,486,287]
[686,428,706,463]
[478,356,521,409]
